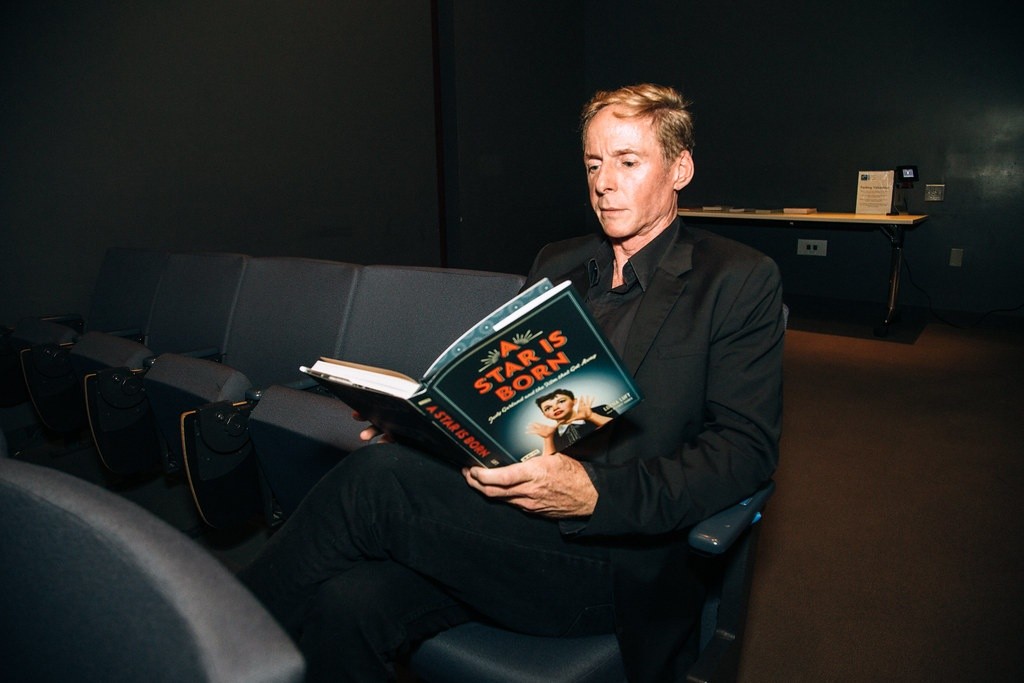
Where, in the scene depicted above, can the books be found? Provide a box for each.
[299,277,643,470]
[677,206,817,215]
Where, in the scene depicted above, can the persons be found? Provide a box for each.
[235,84,786,683]
[528,389,620,455]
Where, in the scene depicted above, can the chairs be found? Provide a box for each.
[0,247,775,683]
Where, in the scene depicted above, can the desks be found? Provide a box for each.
[677,207,930,337]
